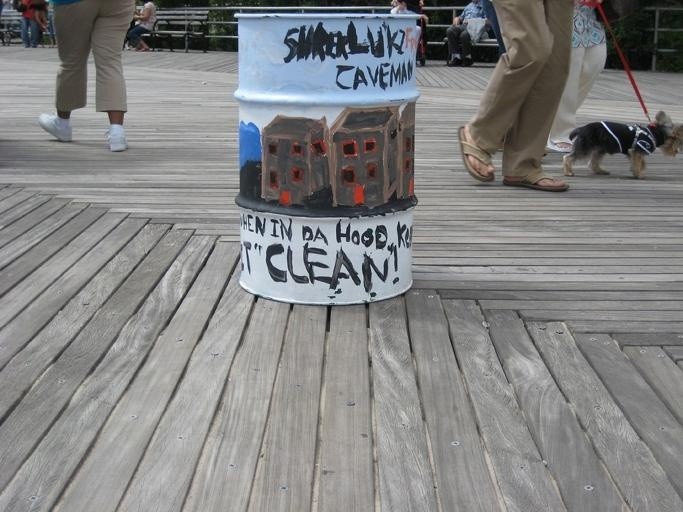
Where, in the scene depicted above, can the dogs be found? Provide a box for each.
[562,110,683,180]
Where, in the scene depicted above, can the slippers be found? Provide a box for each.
[458,126,495,181]
[502,174,569,192]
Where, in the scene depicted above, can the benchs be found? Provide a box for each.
[0,16,54,49]
[140,10,209,53]
[440,36,499,68]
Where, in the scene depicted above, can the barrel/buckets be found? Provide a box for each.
[234,14,422,306]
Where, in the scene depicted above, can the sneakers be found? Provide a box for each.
[105,127,128,152]
[38,111,74,142]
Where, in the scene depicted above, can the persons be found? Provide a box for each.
[0,0,58,48]
[36,0,136,152]
[546,1,608,153]
[390,0,429,58]
[445,0,491,67]
[123,0,158,52]
[458,0,575,192]
